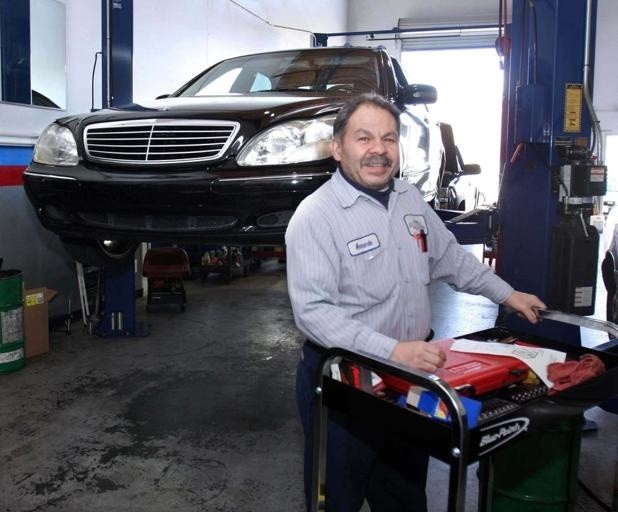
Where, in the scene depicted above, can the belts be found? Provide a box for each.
[306,330,436,363]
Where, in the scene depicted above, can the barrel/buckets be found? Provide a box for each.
[0,268,26,373]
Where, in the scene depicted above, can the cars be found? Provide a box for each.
[21,46,450,272]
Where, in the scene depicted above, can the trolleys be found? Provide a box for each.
[146,278,187,313]
[308,306,618,512]
[196,250,262,283]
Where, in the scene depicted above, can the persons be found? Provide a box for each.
[284,93,546,512]
